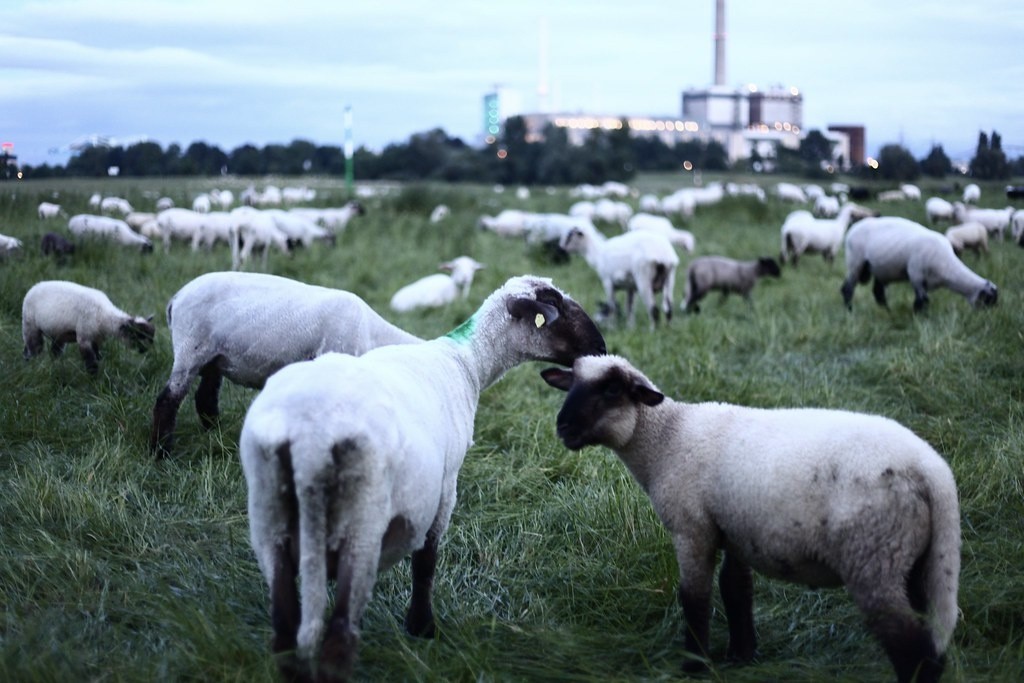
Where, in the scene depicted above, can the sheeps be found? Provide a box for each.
[388,254,483,317]
[0,182,368,273]
[427,181,1024,335]
[539,352,962,682]
[150,270,430,461]
[237,273,611,683]
[21,280,156,378]
